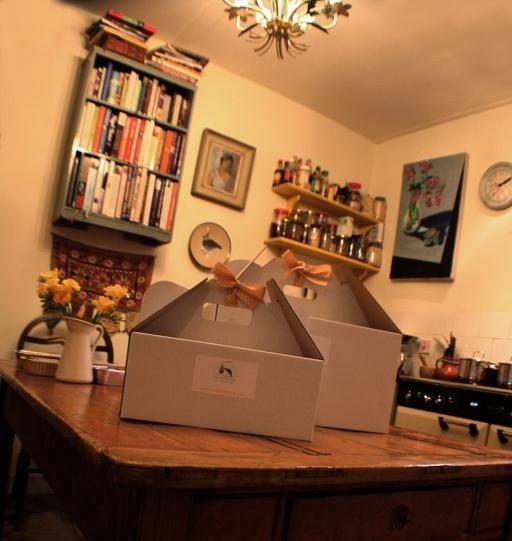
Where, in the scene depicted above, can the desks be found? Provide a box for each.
[0,358,512,540]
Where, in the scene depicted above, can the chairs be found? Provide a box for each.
[6,314,115,533]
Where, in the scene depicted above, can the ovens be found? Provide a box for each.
[391,380,512,455]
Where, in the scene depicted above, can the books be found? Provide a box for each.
[64,8,210,236]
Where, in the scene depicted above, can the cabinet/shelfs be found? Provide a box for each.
[264,181,382,273]
[52,45,198,246]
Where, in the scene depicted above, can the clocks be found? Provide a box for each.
[478,161,512,211]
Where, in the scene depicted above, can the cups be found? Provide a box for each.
[435,358,460,380]
[419,367,434,379]
[457,357,511,389]
[406,354,421,379]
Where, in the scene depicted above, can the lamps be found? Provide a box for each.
[223,0,354,61]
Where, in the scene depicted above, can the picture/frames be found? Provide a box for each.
[190,126,257,211]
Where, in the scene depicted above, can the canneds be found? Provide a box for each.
[373,196,385,220]
[269,207,383,268]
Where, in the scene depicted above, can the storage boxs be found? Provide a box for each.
[115,261,329,443]
[224,241,406,437]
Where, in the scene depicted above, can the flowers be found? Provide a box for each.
[35,268,125,339]
[404,159,447,227]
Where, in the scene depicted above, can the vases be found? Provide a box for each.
[402,196,423,233]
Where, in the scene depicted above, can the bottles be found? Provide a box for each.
[271,156,387,268]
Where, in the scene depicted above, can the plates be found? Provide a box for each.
[190,222,232,271]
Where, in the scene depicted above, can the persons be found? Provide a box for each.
[207,145,235,193]
